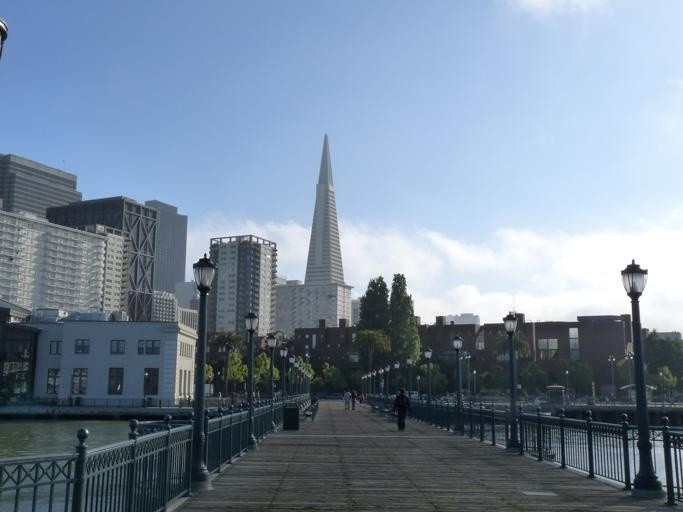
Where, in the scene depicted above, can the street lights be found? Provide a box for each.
[423,346,438,425]
[619,260,668,499]
[185,251,216,494]
[279,344,314,422]
[501,310,526,455]
[451,334,467,435]
[356,354,415,424]
[266,332,278,435]
[240,310,264,454]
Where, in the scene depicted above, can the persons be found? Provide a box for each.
[310,392,319,421]
[391,388,411,431]
[342,389,366,412]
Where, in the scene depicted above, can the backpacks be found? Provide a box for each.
[399,398,407,408]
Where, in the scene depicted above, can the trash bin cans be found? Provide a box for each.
[283,402,299,429]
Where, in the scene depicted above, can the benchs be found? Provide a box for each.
[297,394,319,422]
